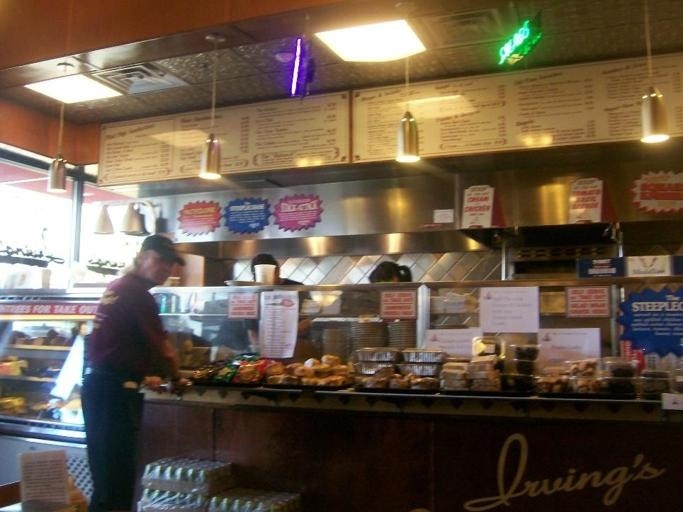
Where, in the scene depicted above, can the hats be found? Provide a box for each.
[143,236,184,266]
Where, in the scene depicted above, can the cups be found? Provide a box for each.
[41,269,50,288]
[254,264,276,285]
[164,276,181,287]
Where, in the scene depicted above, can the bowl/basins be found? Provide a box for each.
[503,338,682,400]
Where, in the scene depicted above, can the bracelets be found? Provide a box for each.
[168,376,180,382]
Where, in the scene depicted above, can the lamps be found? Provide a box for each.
[641,1,669,144]
[94,200,156,235]
[48,62,74,193]
[199,32,226,180]
[395,57,419,163]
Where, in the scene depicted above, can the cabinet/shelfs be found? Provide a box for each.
[0,294,101,504]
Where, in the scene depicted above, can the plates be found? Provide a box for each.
[429,296,466,313]
[223,280,256,286]
[322,320,414,361]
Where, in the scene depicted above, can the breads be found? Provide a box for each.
[265,354,357,387]
[11,329,71,346]
[0,354,29,377]
[0,396,27,416]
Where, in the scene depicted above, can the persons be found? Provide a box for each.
[368,260,411,283]
[80,234,188,511]
[244,252,317,360]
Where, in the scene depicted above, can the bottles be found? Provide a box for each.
[138,457,301,511]
